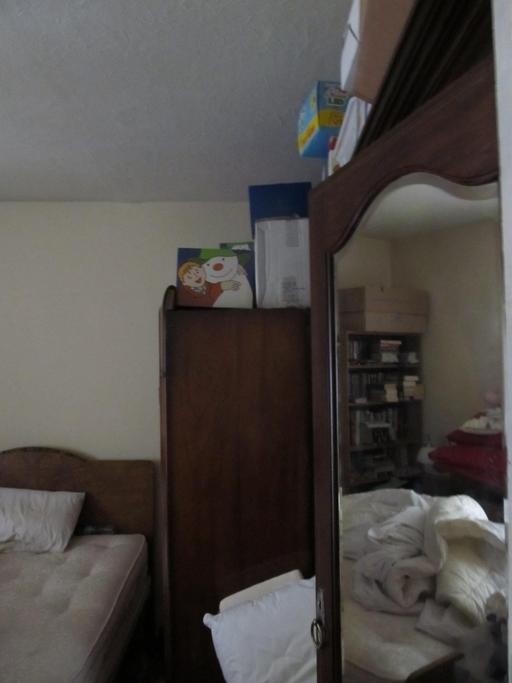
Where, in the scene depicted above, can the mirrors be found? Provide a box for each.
[329,174,509,682]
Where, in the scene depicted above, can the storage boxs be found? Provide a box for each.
[299,81,351,160]
[337,283,429,334]
[344,0,415,104]
[175,181,309,310]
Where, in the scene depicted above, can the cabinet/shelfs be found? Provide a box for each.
[342,329,423,493]
[310,0,512,682]
[159,281,318,683]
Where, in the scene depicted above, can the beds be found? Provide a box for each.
[0,446,160,682]
[336,487,512,683]
[429,440,505,502]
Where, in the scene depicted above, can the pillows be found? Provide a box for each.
[0,484,87,556]
[204,567,318,683]
[444,407,503,446]
